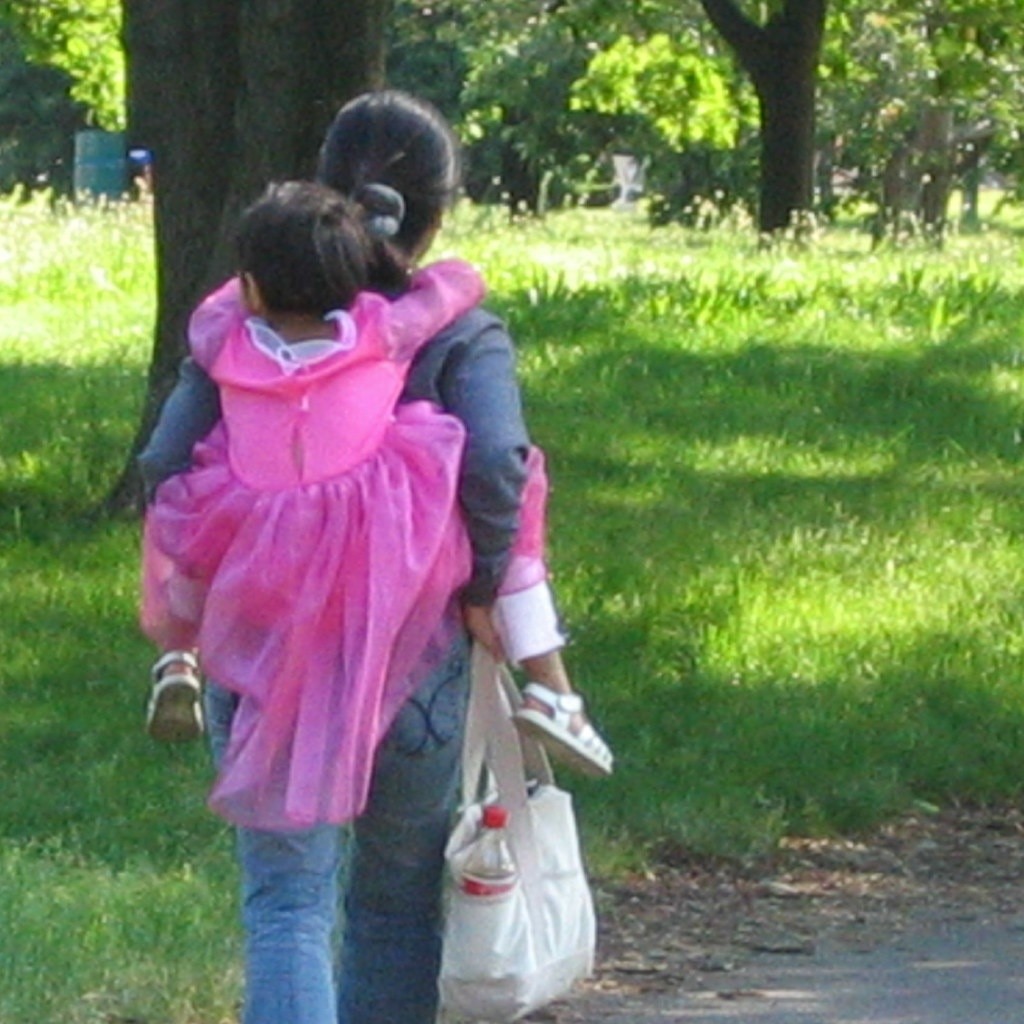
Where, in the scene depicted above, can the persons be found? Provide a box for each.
[127,171,618,781]
[128,90,525,1020]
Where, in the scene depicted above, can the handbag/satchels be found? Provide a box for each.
[436,638,596,1021]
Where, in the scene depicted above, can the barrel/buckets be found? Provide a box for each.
[74,129,127,201]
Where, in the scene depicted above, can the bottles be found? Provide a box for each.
[448,802,516,892]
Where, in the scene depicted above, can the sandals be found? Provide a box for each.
[515,681,614,776]
[145,649,204,744]
